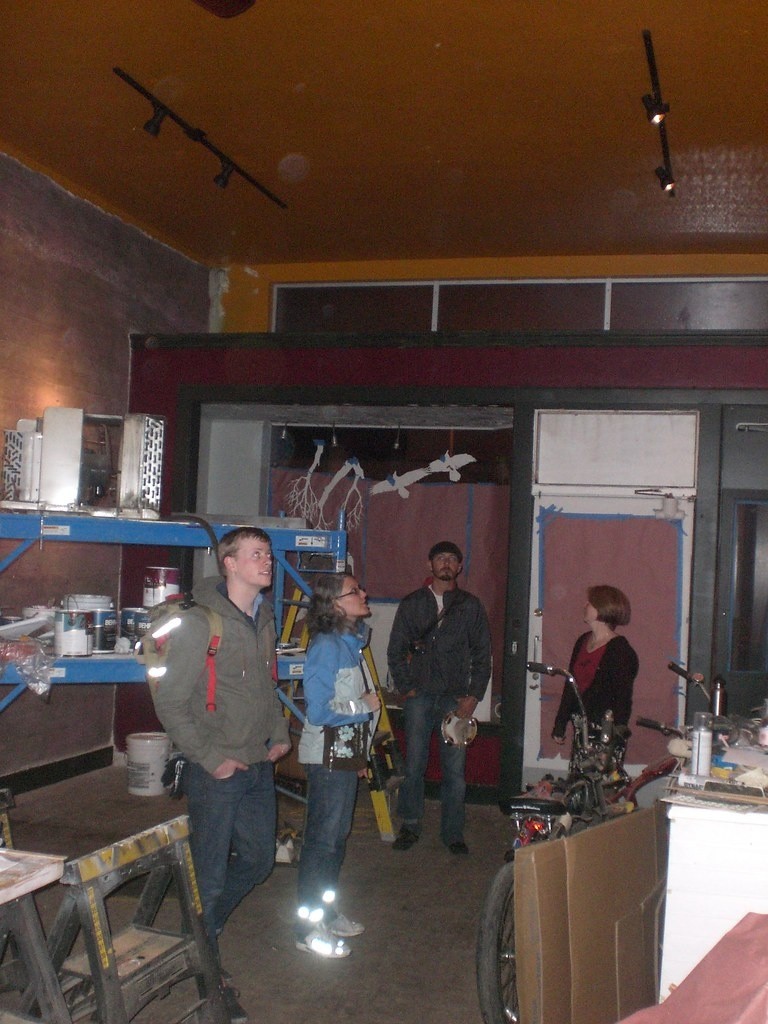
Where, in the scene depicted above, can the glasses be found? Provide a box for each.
[333,586,364,601]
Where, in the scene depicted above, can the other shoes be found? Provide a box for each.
[448,842,470,855]
[391,824,422,851]
[324,913,365,937]
[219,971,248,1023]
[295,929,352,958]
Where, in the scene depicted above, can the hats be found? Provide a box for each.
[429,541,461,555]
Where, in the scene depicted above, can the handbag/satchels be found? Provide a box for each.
[320,719,372,770]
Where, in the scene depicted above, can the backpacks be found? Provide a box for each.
[141,597,270,726]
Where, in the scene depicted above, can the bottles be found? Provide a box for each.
[692,712,713,778]
[711,675,727,723]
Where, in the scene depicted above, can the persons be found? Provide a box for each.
[549,585,640,775]
[294,571,381,960]
[155,526,291,1024]
[386,541,492,857]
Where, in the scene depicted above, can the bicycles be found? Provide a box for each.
[473,662,735,1024]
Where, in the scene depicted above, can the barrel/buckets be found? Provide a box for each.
[124,732,171,796]
[142,566,180,610]
[0,605,60,654]
[121,607,158,655]
[54,595,94,657]
[63,593,117,653]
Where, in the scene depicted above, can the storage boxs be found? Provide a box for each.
[370,776,405,843]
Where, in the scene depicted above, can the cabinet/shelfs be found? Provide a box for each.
[0,513,351,683]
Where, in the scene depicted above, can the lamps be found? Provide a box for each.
[215,163,232,188]
[331,421,338,447]
[282,420,287,439]
[642,94,667,124]
[393,421,401,449]
[143,109,171,136]
[655,166,675,191]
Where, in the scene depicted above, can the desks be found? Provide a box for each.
[659,797,768,1007]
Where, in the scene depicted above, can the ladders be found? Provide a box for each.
[272,549,402,842]
